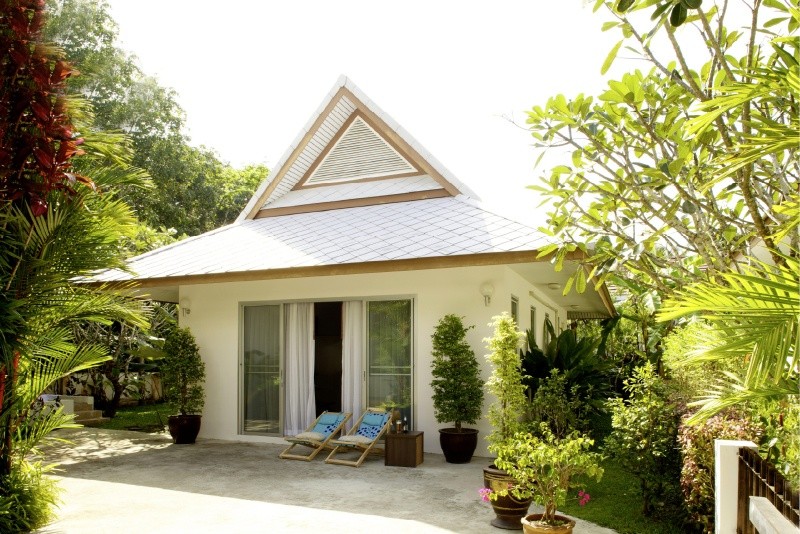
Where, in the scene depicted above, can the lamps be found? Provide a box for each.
[479,280,496,307]
[179,296,192,318]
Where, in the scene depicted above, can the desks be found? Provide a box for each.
[382,428,426,469]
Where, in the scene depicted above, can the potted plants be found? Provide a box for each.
[483,418,610,534]
[156,324,208,447]
[429,313,486,465]
[481,310,538,531]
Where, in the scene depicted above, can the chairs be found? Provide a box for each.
[279,409,354,463]
[324,407,395,468]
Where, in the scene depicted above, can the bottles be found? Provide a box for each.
[403,416,408,434]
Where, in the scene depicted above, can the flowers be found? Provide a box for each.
[477,485,495,505]
[574,487,591,506]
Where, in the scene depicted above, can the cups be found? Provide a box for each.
[395,419,402,434]
